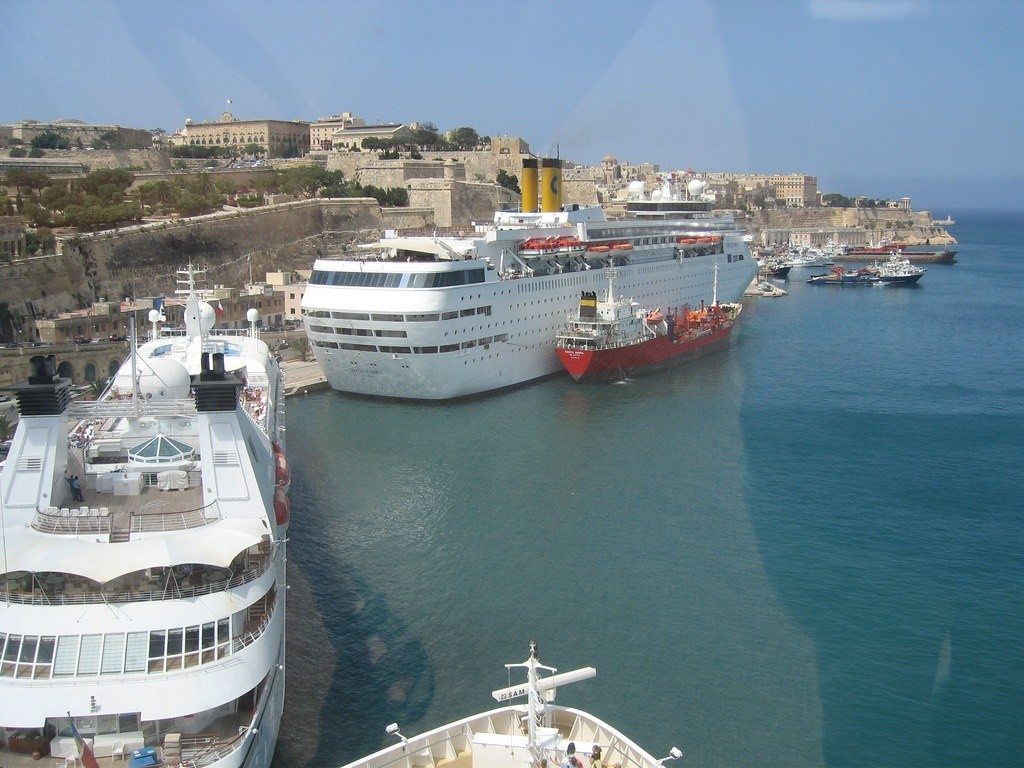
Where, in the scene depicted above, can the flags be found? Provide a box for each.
[69,717,100,768]
[158,302,167,321]
[217,301,224,316]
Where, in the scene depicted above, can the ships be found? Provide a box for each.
[299,158,766,400]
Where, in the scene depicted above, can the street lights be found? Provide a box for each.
[123,325,126,341]
[19,330,22,352]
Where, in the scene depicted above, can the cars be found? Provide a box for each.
[0,342,23,348]
[91,338,106,343]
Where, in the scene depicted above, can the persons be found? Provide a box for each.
[589,745,608,768]
[614,761,621,768]
[538,759,547,768]
[560,742,584,768]
[67,426,89,448]
[245,386,264,417]
[65,475,85,502]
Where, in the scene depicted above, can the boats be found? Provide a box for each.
[694,238,712,250]
[555,237,587,258]
[582,245,611,260]
[753,235,959,286]
[272,487,291,539]
[332,636,685,768]
[518,239,559,260]
[609,244,633,258]
[273,443,292,494]
[676,239,696,251]
[711,237,720,244]
[0,252,293,768]
[551,258,745,383]
